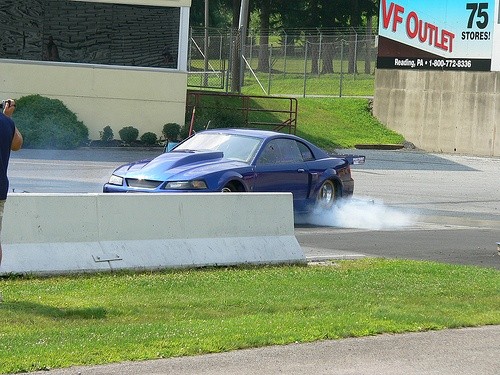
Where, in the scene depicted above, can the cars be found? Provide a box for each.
[100,128,355,224]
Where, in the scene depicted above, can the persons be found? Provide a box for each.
[0,99,23,264]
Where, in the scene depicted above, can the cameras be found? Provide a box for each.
[0,100,11,109]
